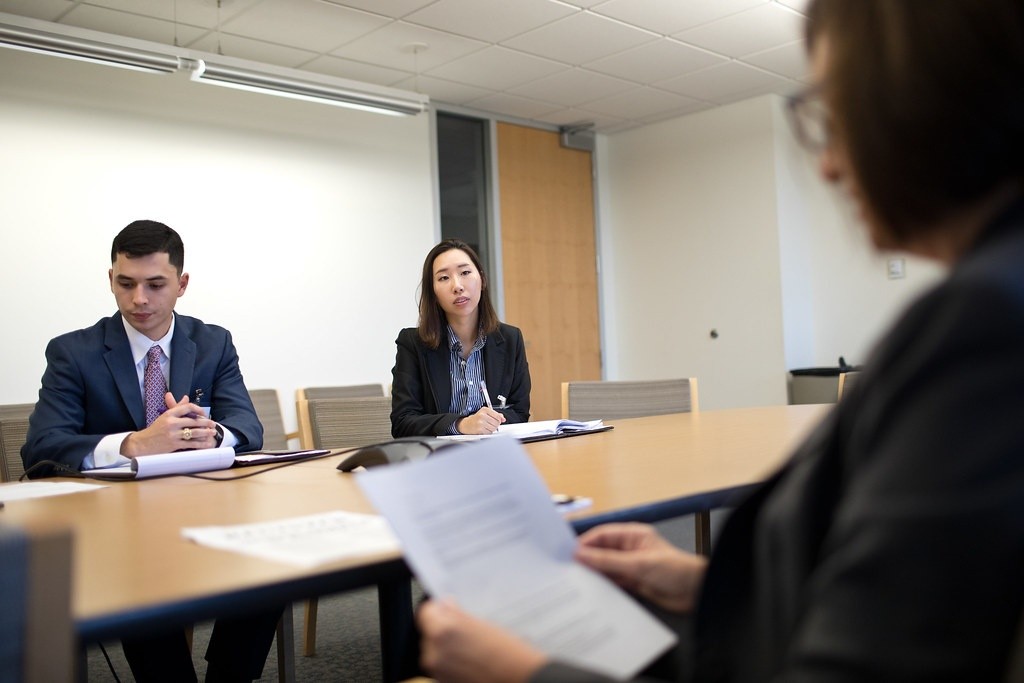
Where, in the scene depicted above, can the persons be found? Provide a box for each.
[408,1,1024,682]
[390,238,532,437]
[19,219,284,682]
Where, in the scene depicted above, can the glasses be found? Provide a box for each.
[784,82,842,152]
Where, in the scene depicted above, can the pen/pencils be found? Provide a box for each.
[481,380,498,431]
[157,408,197,419]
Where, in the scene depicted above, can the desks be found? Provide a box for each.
[2,407,832,683]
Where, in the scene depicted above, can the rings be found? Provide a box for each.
[184,428,191,440]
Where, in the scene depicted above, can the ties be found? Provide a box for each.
[146,345,168,428]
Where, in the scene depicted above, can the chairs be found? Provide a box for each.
[0,402,196,683]
[294,384,395,657]
[241,390,298,683]
[561,377,712,558]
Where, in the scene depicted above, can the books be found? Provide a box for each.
[438,420,615,447]
[79,446,235,481]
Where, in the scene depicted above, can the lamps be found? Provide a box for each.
[192,0,430,119]
[0,0,183,74]
